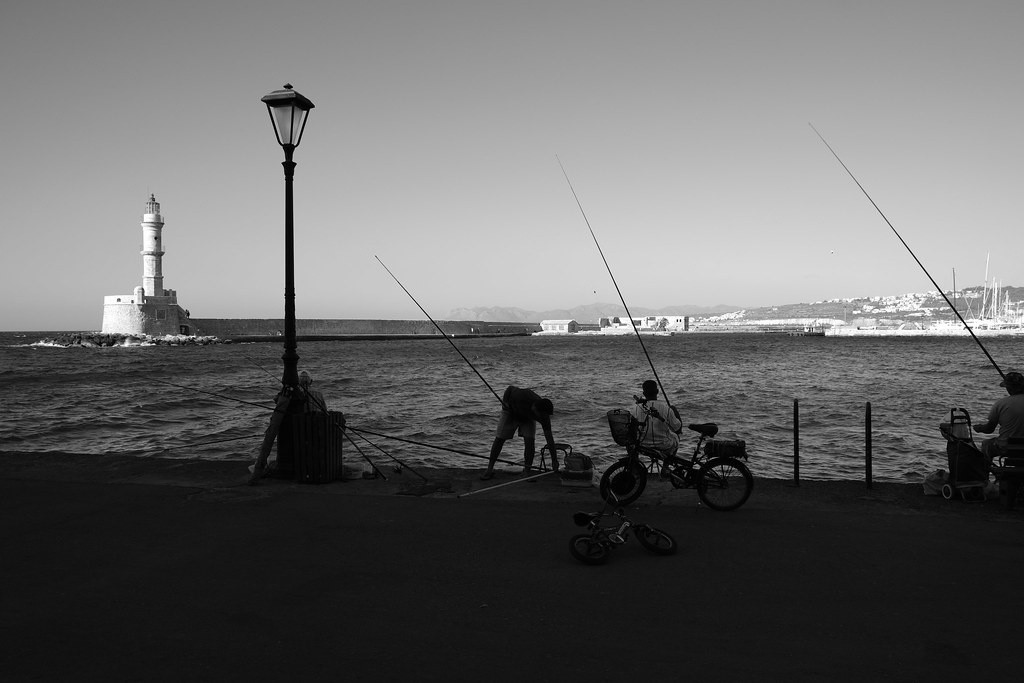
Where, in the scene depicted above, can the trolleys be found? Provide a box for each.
[938,406,990,504]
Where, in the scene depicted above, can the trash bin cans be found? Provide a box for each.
[294,410,346,485]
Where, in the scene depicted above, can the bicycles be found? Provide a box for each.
[598,393,755,512]
[568,485,683,566]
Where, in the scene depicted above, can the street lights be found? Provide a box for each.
[259,80,316,415]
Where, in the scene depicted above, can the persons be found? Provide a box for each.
[974,372,1024,467]
[295,368,329,415]
[482,386,560,483]
[631,378,684,480]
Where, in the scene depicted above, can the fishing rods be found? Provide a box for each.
[808,121,1005,380]
[555,154,684,436]
[101,253,559,494]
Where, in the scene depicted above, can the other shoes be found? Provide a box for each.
[522,469,536,482]
[480,469,495,479]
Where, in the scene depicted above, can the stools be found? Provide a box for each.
[539,443,573,473]
[994,447,1024,492]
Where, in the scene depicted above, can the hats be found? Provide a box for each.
[1000,372,1024,387]
